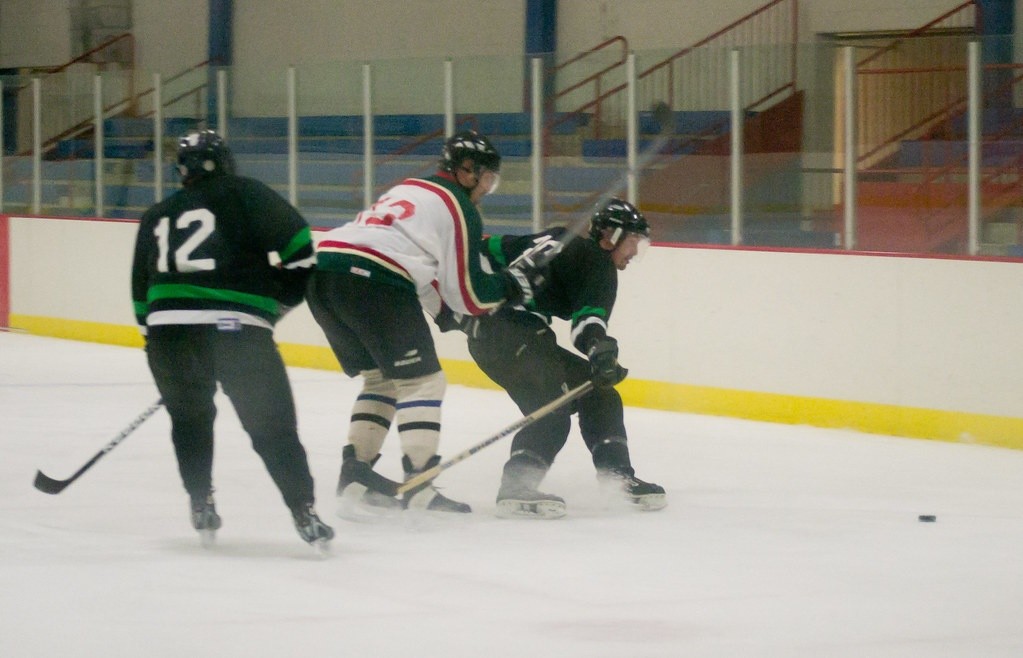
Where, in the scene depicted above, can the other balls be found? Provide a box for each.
[919,515,935,522]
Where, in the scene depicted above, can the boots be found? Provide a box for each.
[400,452,474,525]
[590,435,666,512]
[492,449,567,519]
[330,445,403,526]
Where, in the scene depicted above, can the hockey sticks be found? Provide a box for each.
[397,367,629,494]
[35,398,164,495]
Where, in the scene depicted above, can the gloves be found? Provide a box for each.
[587,335,629,391]
[439,304,490,340]
[505,264,553,302]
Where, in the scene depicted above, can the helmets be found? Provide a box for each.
[436,128,502,173]
[174,130,236,185]
[588,197,652,238]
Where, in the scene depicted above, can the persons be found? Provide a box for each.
[132,127,336,545]
[303,129,551,512]
[49,127,116,217]
[466,195,666,508]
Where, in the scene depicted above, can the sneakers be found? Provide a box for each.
[190,491,222,546]
[293,500,336,559]
